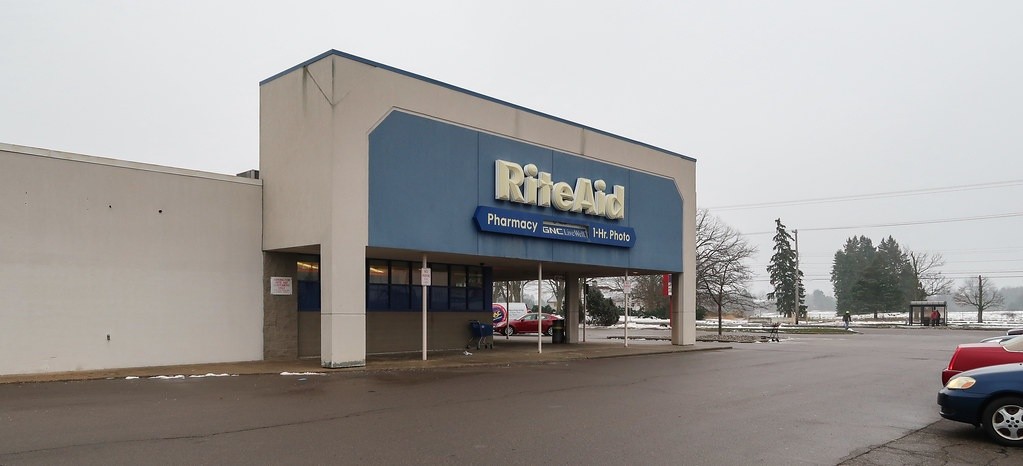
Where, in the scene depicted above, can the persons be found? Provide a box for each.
[931,310,941,326]
[842,311,851,330]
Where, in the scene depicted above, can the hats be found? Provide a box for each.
[846,311,849,314]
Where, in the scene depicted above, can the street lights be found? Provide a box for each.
[792,230,798,324]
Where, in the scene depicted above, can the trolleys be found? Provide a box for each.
[467,319,493,350]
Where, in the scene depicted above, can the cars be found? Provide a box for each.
[942,332,1023,387]
[936,362,1023,449]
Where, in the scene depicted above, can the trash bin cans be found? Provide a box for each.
[551,319,565,344]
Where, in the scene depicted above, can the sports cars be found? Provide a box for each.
[493,313,564,335]
